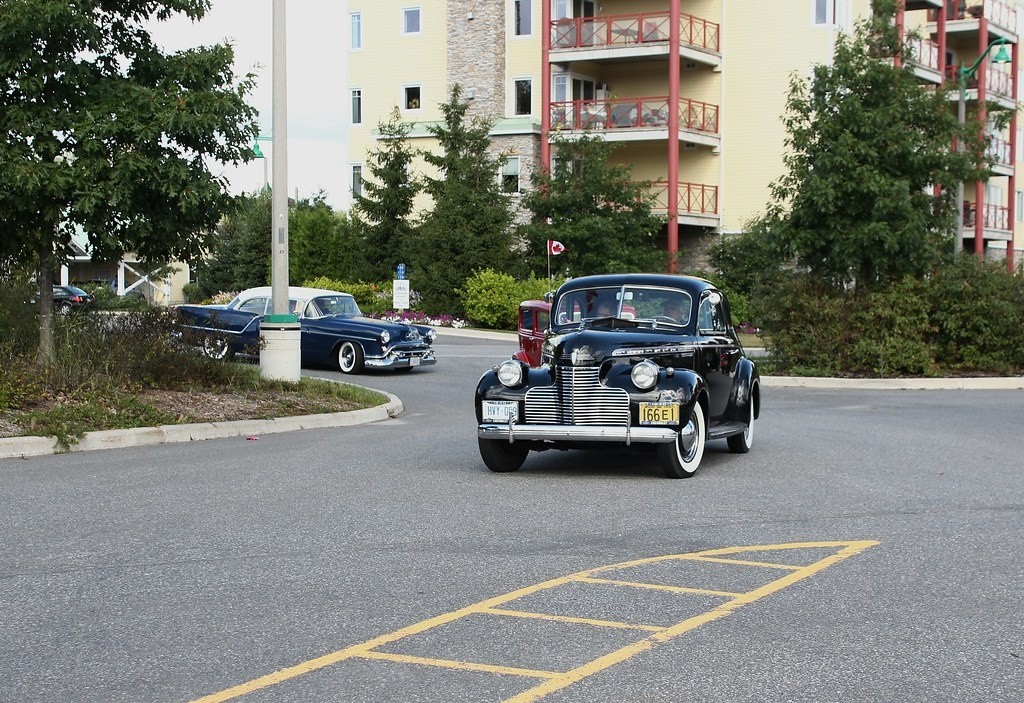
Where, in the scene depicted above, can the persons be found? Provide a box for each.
[588,299,616,329]
[90,288,98,303]
[659,299,689,325]
[315,298,332,315]
[110,274,118,293]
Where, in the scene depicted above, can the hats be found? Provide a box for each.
[660,298,685,307]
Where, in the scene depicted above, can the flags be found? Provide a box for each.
[547,242,571,256]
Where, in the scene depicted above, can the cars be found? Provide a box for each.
[31,283,94,317]
[509,295,636,369]
[473,272,762,480]
[167,286,438,375]
[86,277,146,304]
